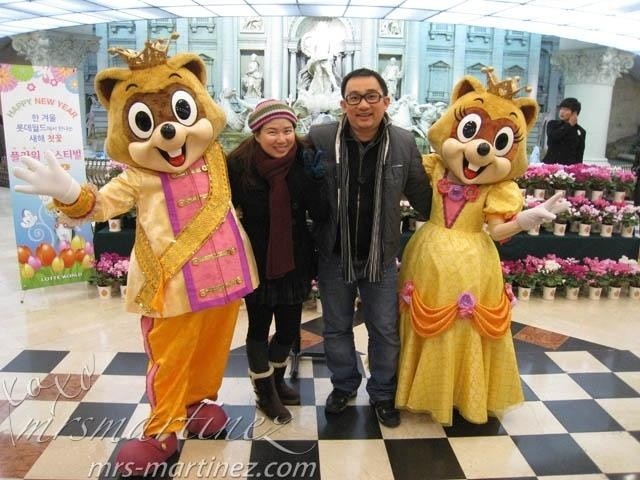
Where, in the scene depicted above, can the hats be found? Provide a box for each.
[247,97,298,134]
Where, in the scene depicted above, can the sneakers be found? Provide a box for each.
[369,394,401,429]
[324,387,358,416]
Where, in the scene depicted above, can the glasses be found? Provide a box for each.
[342,90,384,106]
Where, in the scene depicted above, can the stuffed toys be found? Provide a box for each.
[392,65,568,425]
[11,31,261,474]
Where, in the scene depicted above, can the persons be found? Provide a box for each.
[241,53,263,100]
[311,109,338,125]
[226,98,332,425]
[388,94,447,137]
[382,56,403,97]
[305,67,433,427]
[541,97,587,165]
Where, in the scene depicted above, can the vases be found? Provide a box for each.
[414,221,427,232]
[316,297,323,314]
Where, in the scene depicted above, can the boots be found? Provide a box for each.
[248,363,293,424]
[268,360,301,405]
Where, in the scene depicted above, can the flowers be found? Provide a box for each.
[310,278,318,301]
[87,159,137,301]
[499,159,640,305]
[398,198,420,221]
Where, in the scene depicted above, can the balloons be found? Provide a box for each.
[17,236,94,280]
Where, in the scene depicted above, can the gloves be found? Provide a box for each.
[301,148,325,180]
